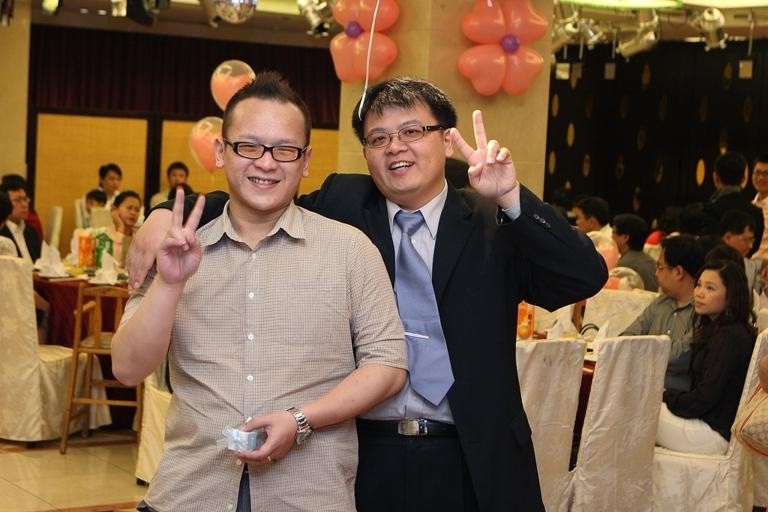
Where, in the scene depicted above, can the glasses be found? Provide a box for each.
[363,125,444,148]
[223,138,308,162]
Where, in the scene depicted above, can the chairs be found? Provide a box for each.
[515,340,587,512]
[134,371,186,490]
[583,288,664,339]
[653,330,764,512]
[572,334,671,512]
[535,297,578,338]
[60,281,143,456]
[2,254,112,448]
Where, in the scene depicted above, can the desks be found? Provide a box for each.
[32,260,136,344]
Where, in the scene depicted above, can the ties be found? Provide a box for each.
[395,211,455,407]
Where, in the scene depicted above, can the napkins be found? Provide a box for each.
[547,315,581,341]
[95,251,117,285]
[35,253,66,277]
[593,320,612,356]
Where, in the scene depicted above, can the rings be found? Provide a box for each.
[266,453,274,466]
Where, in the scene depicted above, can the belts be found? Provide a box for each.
[356,419,459,437]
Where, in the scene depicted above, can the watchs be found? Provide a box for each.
[286,406,313,447]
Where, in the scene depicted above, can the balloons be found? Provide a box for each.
[188,116,225,176]
[328,0,402,85]
[457,1,549,96]
[210,59,256,113]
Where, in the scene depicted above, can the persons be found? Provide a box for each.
[126,76,611,512]
[0,159,194,346]
[112,70,409,512]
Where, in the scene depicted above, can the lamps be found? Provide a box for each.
[0,0,339,42]
[546,0,729,61]
